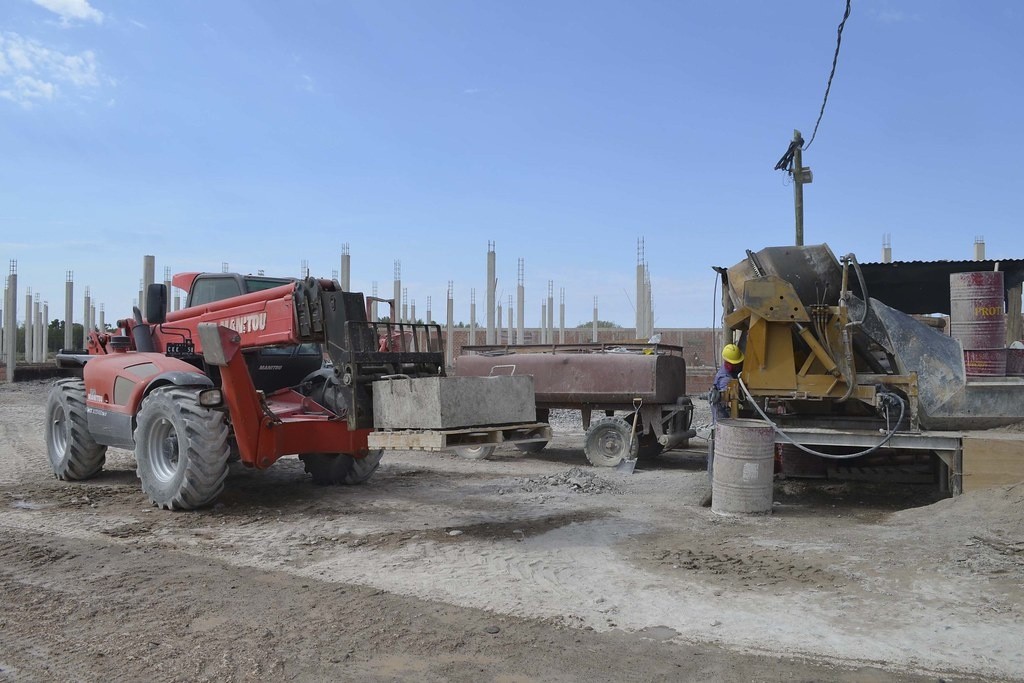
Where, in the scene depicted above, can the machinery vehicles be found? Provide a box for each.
[42,269,551,513]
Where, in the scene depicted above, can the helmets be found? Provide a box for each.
[721,343,744,365]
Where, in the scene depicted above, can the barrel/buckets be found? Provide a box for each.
[949,272,1005,350]
[711,418,777,518]
[964,313,1008,377]
[1007,348,1024,375]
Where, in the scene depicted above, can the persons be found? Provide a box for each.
[710,344,745,421]
